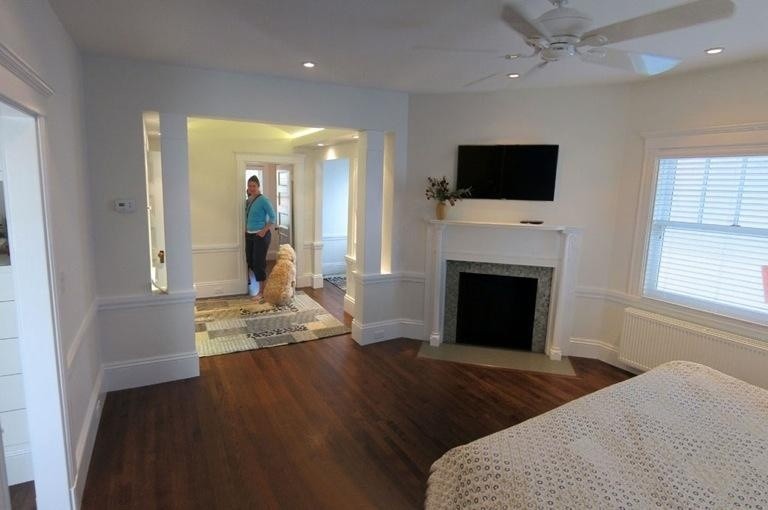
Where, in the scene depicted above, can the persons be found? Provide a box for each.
[245,175,276,301]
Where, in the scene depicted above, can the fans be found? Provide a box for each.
[414,0,734,88]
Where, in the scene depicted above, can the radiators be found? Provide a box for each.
[617,307,768,391]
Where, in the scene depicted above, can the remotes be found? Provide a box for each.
[520,221,544,224]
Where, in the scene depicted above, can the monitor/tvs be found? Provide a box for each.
[456,144,559,201]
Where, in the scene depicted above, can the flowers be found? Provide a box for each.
[424,176,472,206]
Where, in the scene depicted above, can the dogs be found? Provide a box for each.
[263,244,296,306]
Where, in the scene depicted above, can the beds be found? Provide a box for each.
[425,360,768,510]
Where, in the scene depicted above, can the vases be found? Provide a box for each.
[435,200,447,219]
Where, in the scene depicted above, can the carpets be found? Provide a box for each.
[418,342,576,376]
[324,277,346,292]
[195,290,352,357]
